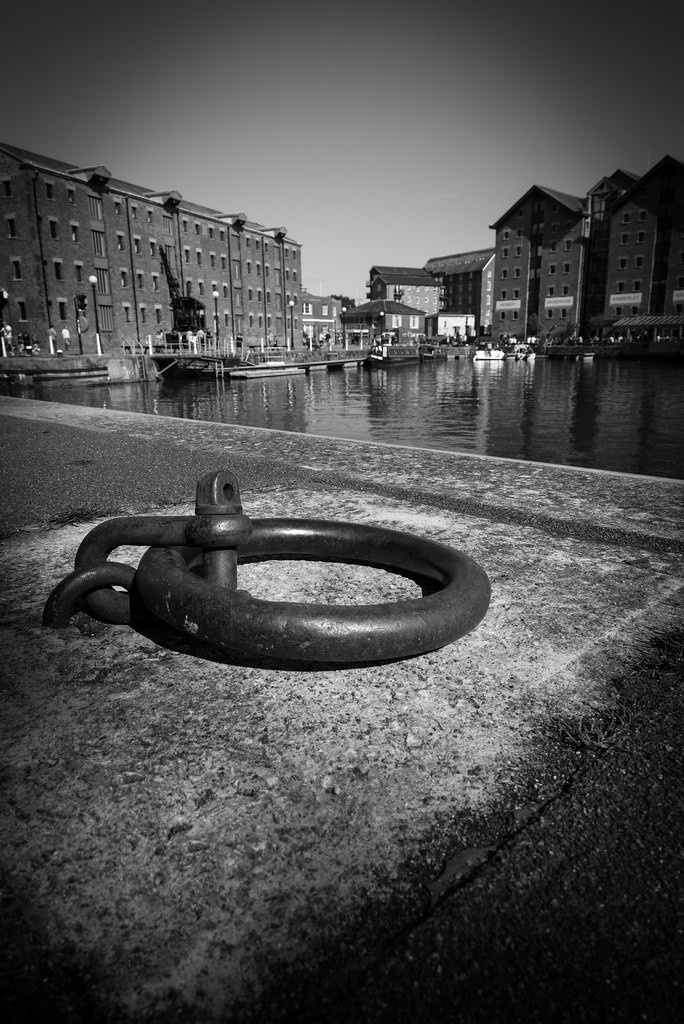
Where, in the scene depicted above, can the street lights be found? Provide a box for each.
[380,311,384,334]
[289,301,295,350]
[89,275,104,353]
[341,306,346,349]
[212,291,219,350]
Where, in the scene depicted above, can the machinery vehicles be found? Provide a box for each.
[157,243,212,350]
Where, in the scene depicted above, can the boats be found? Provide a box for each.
[370,331,419,364]
[471,337,506,361]
[506,344,536,360]
[228,347,307,376]
[550,352,596,361]
[410,333,448,360]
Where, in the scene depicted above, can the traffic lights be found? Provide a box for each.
[77,295,87,310]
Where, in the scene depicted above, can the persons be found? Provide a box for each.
[455,332,462,346]
[574,334,623,346]
[502,331,539,346]
[236,333,243,350]
[319,332,326,345]
[17,332,25,354]
[62,325,70,350]
[155,329,167,353]
[325,332,331,347]
[46,324,58,353]
[31,333,40,354]
[0,321,15,356]
[268,330,274,347]
[443,333,447,336]
